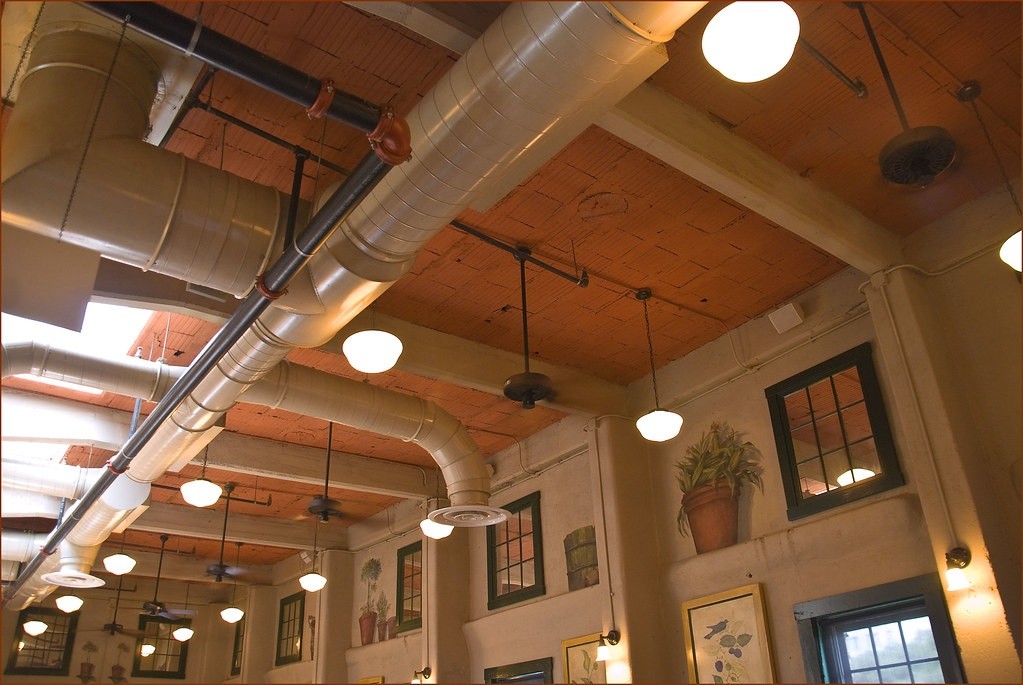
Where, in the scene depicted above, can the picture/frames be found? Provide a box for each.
[562,631,606,685]
[681,583,777,685]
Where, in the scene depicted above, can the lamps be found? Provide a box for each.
[702,0,800,83]
[945,547,971,592]
[635,287,683,443]
[341,303,403,374]
[595,630,620,661]
[19,418,453,657]
[956,80,1023,272]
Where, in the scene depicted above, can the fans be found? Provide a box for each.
[176,482,275,588]
[62,529,146,640]
[273,422,391,531]
[840,1,956,186]
[455,246,607,429]
[111,534,197,624]
